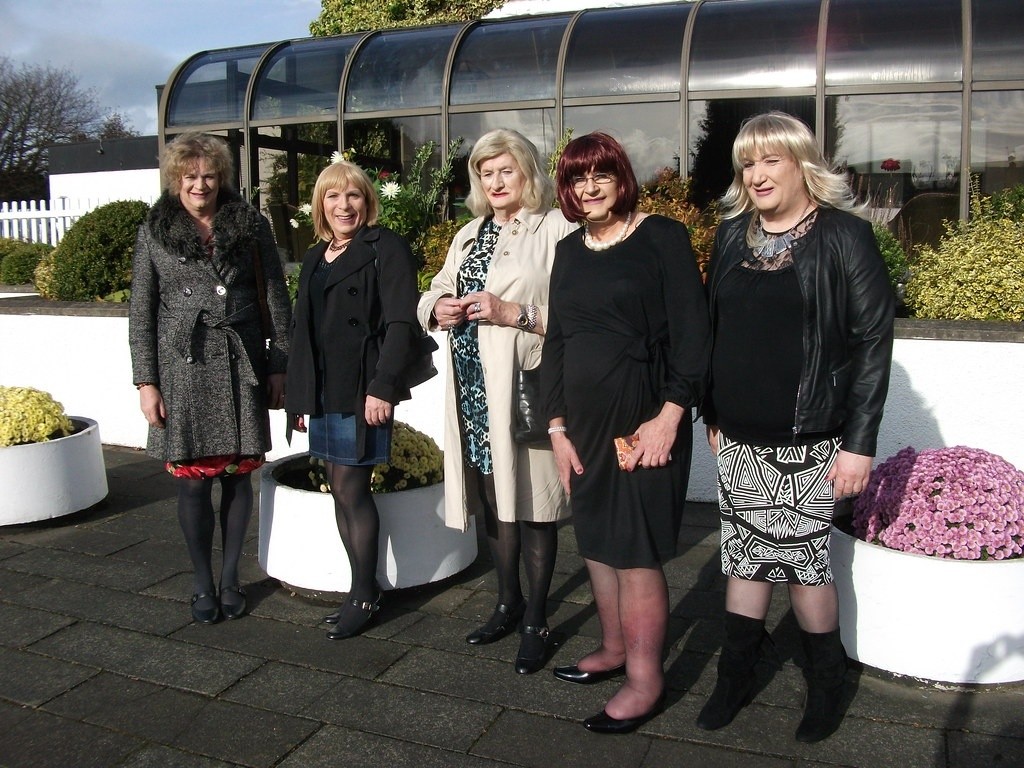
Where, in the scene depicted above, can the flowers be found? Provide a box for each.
[308,420,445,495]
[881,157,901,186]
[0,384,76,448]
[845,445,1024,561]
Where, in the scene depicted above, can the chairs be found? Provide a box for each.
[898,193,960,261]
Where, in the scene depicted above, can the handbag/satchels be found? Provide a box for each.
[372,240,439,388]
[514,363,552,444]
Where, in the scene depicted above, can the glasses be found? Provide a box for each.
[574,174,617,188]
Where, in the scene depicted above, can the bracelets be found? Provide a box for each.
[137,382,151,390]
[547,426,567,434]
[527,305,537,332]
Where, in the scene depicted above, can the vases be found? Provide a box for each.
[884,188,898,209]
[0,416,109,530]
[257,450,479,602]
[831,497,1024,685]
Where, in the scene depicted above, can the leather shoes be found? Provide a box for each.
[324,591,384,640]
[553,665,626,685]
[515,625,550,674]
[190,584,219,625]
[584,688,667,734]
[220,586,247,619]
[466,599,527,644]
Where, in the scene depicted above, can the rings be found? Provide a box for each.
[474,303,482,319]
[447,321,452,327]
[282,394,286,397]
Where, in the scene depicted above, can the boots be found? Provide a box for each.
[795,627,855,742]
[697,612,766,730]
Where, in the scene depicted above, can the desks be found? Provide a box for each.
[869,207,902,240]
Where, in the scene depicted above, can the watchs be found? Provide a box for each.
[517,303,529,330]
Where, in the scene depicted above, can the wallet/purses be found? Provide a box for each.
[614,434,672,471]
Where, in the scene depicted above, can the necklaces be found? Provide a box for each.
[584,210,631,251]
[330,236,352,253]
[754,199,810,257]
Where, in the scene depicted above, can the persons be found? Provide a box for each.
[417,128,583,673]
[692,111,894,744]
[540,131,713,734]
[284,161,422,641]
[129,132,293,625]
